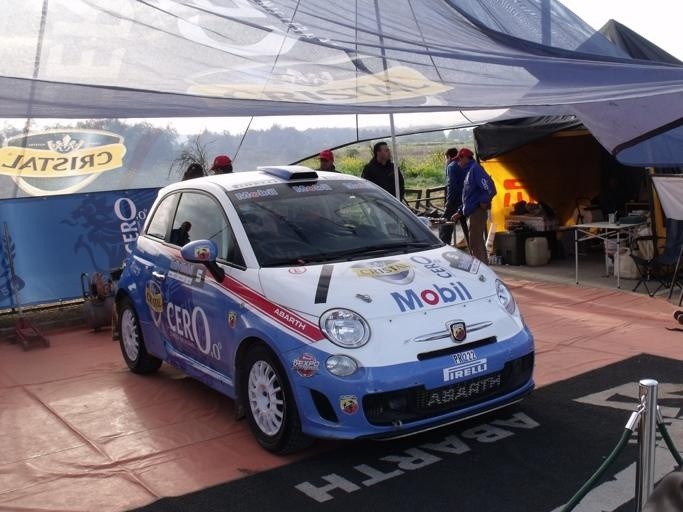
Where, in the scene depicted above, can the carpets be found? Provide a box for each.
[127,352,683,512]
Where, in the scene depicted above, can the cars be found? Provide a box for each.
[115,166,535,458]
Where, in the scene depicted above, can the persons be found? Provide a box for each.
[315,150,340,174]
[359,140,405,203]
[207,155,232,176]
[438,147,468,244]
[451,147,497,264]
[180,163,203,182]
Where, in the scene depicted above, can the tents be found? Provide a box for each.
[473,17,683,280]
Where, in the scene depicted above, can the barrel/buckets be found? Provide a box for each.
[525,237,549,266]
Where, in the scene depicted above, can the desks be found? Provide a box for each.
[571,221,647,288]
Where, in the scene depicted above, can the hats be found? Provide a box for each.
[318,150,333,160]
[210,156,231,171]
[453,149,473,159]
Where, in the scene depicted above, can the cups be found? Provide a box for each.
[608,214,614,224]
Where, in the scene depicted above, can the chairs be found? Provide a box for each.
[630,239,683,297]
